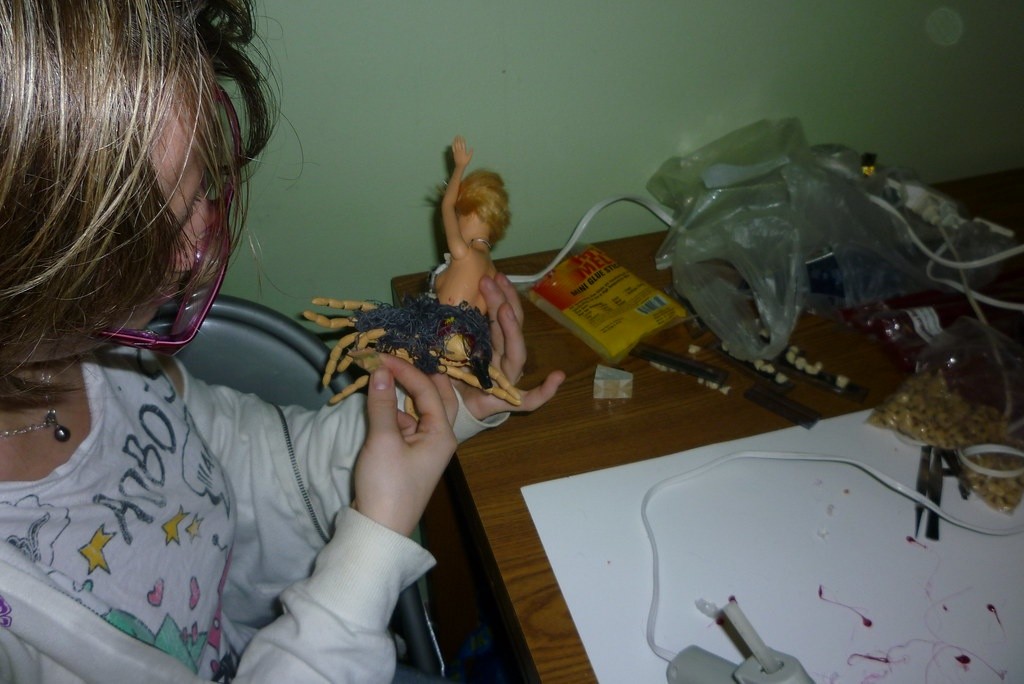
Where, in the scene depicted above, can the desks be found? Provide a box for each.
[391,168,1023,683]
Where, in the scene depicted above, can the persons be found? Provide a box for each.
[0,0,568,684]
[434,134,511,317]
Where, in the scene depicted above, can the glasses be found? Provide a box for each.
[96,83,242,359]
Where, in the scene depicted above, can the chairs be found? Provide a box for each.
[145,290,453,683]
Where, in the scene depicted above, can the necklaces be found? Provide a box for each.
[0,367,71,442]
